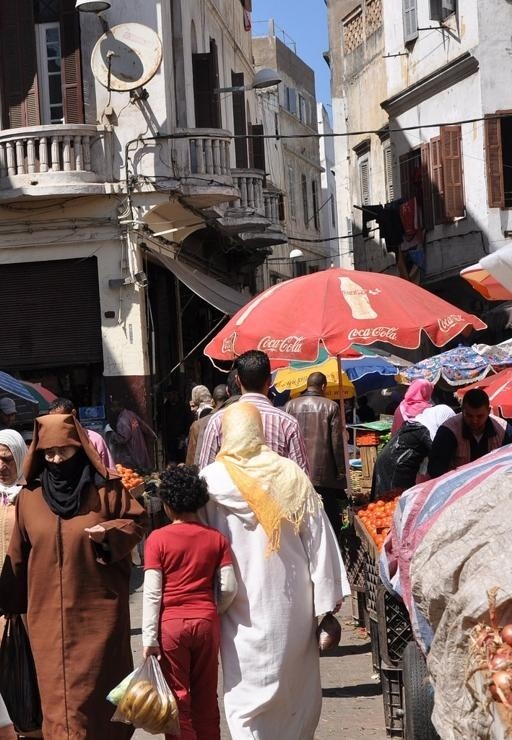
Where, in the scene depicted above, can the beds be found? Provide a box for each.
[204,261,488,501]
[266,343,415,401]
[454,366,511,420]
[396,341,512,390]
[1,371,59,413]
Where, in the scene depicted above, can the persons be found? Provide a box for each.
[48,398,115,478]
[141,460,237,738]
[193,402,354,740]
[1,396,19,427]
[369,402,457,502]
[0,425,41,739]
[185,385,229,465]
[200,350,310,477]
[0,411,150,738]
[391,378,434,442]
[105,396,160,473]
[427,388,512,482]
[195,369,243,464]
[286,372,347,538]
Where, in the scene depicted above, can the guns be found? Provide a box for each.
[121,679,179,735]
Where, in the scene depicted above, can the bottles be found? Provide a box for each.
[0,398,18,415]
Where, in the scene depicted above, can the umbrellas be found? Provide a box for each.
[454,366,511,420]
[1,371,59,413]
[396,341,512,390]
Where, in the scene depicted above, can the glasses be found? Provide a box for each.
[213,68,283,95]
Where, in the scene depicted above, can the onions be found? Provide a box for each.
[489,621,512,704]
